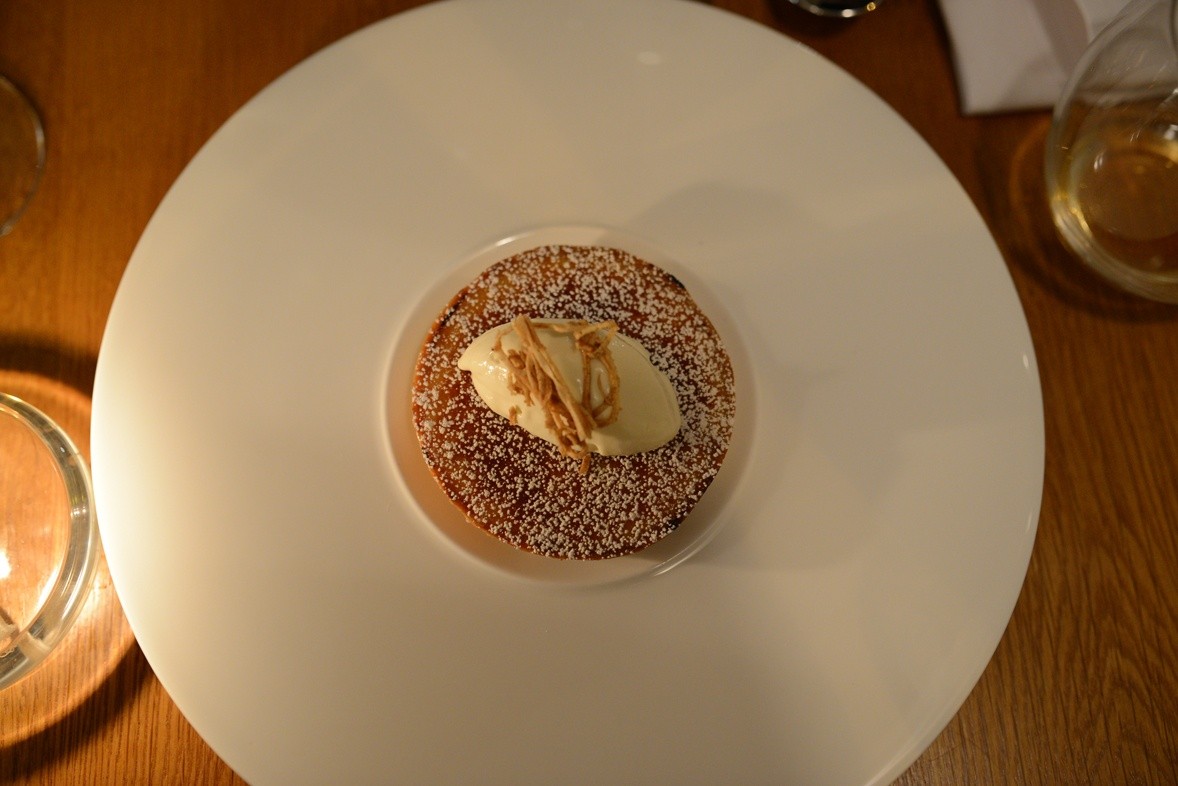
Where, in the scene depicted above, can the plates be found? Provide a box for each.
[92,0,1045,785]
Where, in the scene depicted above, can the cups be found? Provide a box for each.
[3,391,101,696]
[1045,1,1177,306]
